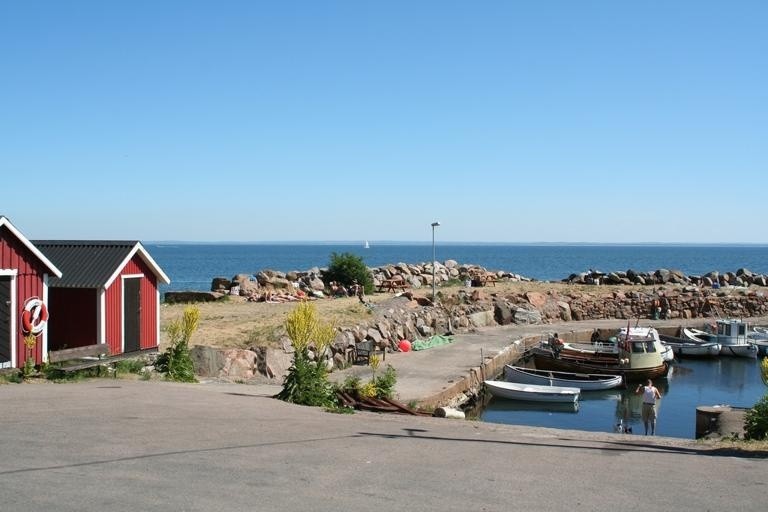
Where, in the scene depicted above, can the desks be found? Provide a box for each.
[378,279,406,293]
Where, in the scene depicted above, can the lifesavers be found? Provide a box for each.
[24,298,49,334]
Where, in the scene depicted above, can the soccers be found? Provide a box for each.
[400,340,411,351]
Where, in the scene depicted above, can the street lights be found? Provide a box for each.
[430,222,441,297]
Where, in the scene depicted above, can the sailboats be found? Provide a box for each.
[363,240,370,248]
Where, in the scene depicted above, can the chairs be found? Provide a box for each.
[330,281,346,297]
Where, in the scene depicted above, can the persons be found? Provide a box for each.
[660,291,673,319]
[591,328,601,342]
[244,279,368,304]
[550,332,563,346]
[651,296,660,320]
[636,379,662,435]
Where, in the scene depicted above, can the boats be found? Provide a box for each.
[477,316,767,405]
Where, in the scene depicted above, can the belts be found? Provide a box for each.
[643,402,656,406]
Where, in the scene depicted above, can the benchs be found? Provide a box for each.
[376,285,409,293]
[355,341,386,366]
[482,280,499,287]
